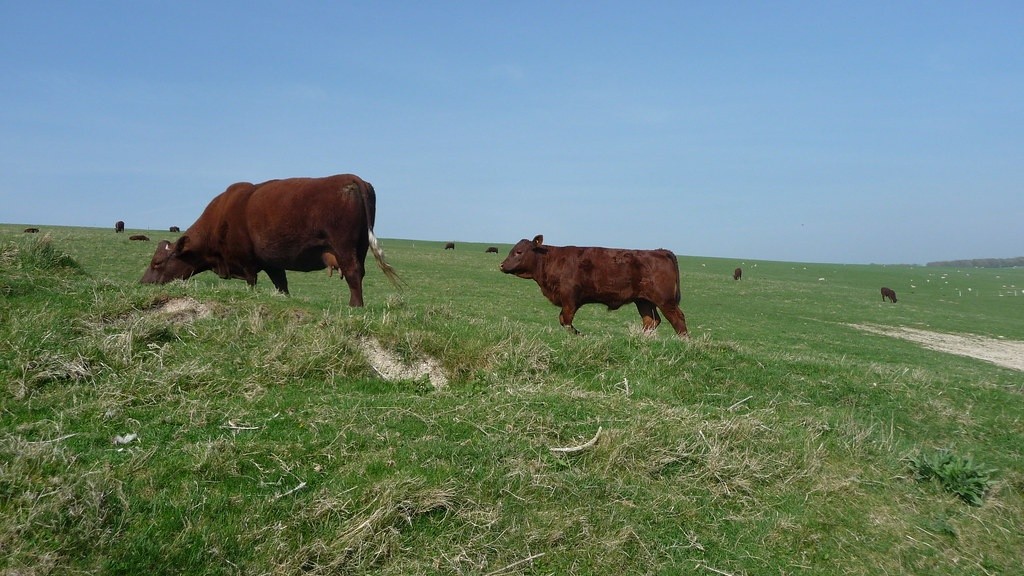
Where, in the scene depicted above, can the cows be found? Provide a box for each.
[732,267,742,281]
[169,226,180,233]
[129,235,150,241]
[499,234,691,341]
[113,220,125,234]
[137,172,410,309]
[24,228,40,234]
[881,287,898,304]
[485,246,499,254]
[444,242,455,250]
